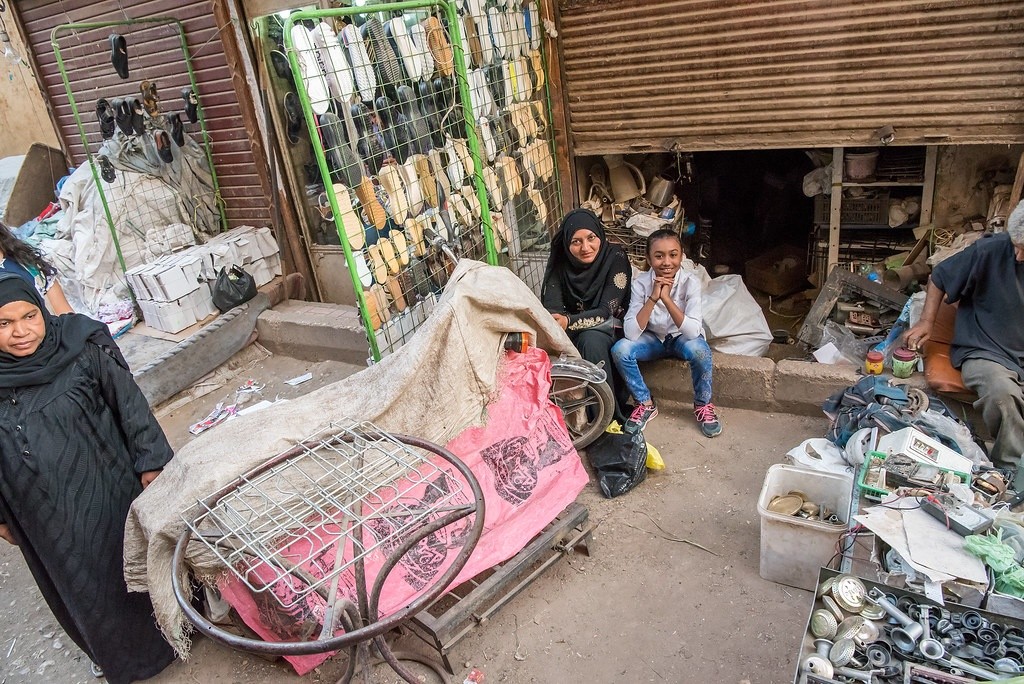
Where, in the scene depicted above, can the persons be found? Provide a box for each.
[541,208,633,431]
[0,219,209,684]
[902,200,1024,489]
[611,229,721,438]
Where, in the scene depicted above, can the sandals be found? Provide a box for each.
[139,81,160,117]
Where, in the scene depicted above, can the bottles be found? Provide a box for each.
[860,263,881,285]
[865,352,884,374]
[891,346,918,378]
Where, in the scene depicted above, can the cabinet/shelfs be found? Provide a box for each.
[827,146,939,282]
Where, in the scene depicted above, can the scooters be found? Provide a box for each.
[422,230,615,453]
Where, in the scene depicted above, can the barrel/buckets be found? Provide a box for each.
[843,148,879,179]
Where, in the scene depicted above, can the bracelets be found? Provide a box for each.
[649,297,657,304]
[565,315,570,327]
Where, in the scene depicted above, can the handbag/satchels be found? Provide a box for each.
[588,315,623,339]
[212,264,258,314]
[606,420,665,470]
[585,428,647,499]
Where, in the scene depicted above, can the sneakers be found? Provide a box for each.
[694,401,722,437]
[625,396,658,435]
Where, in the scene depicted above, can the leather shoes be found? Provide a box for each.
[182,89,198,124]
[125,97,145,135]
[112,99,133,135]
[167,111,184,147]
[109,34,129,79]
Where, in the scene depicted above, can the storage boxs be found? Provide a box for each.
[602,229,649,258]
[793,566,1024,684]
[904,542,1024,619]
[746,244,808,295]
[126,225,281,335]
[756,463,855,593]
[813,188,892,225]
[816,249,884,290]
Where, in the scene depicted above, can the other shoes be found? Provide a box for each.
[97,154,116,183]
[96,99,115,140]
[153,130,173,163]
[268,0,553,366]
[91,662,104,678]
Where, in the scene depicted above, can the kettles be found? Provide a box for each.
[603,155,646,203]
[645,173,675,207]
